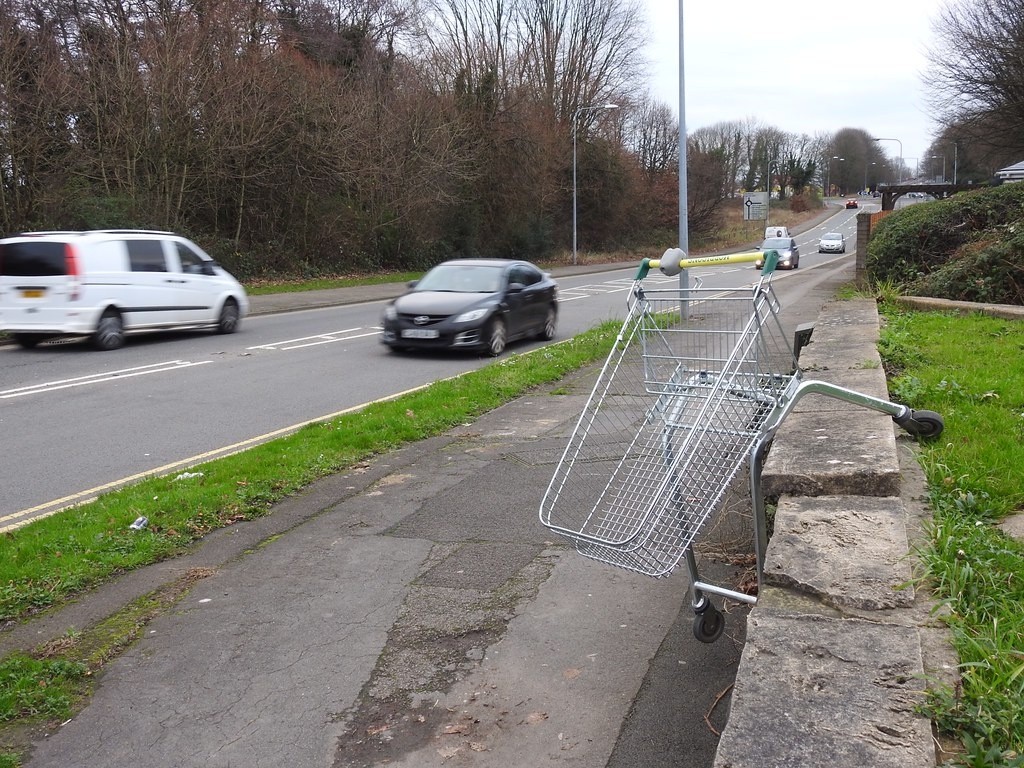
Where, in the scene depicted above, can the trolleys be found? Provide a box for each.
[538,246,945,644]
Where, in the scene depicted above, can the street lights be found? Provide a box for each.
[874,138,903,185]
[932,155,946,182]
[766,159,792,224]
[573,105,619,266]
[827,156,839,197]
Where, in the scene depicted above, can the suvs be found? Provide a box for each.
[764,226,792,239]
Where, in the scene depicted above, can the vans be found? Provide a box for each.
[0,229,246,352]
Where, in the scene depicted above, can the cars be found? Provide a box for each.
[845,198,857,209]
[755,237,798,271]
[380,259,558,358]
[819,232,846,254]
[840,189,882,198]
[906,191,924,199]
